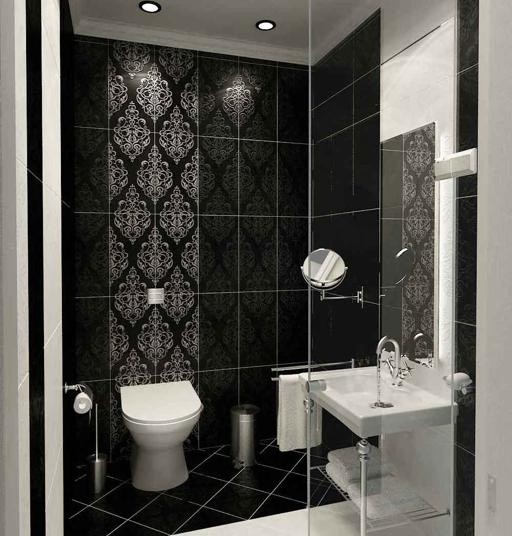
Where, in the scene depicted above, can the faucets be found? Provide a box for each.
[410,330,433,360]
[376,336,401,383]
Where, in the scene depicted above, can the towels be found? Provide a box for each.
[277,373,325,452]
[326,445,421,520]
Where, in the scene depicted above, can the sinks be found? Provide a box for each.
[299,366,461,439]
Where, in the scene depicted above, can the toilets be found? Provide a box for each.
[120,380,204,491]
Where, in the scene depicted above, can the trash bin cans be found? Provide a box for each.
[230,404,260,467]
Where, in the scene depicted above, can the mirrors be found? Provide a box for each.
[379,121,442,370]
